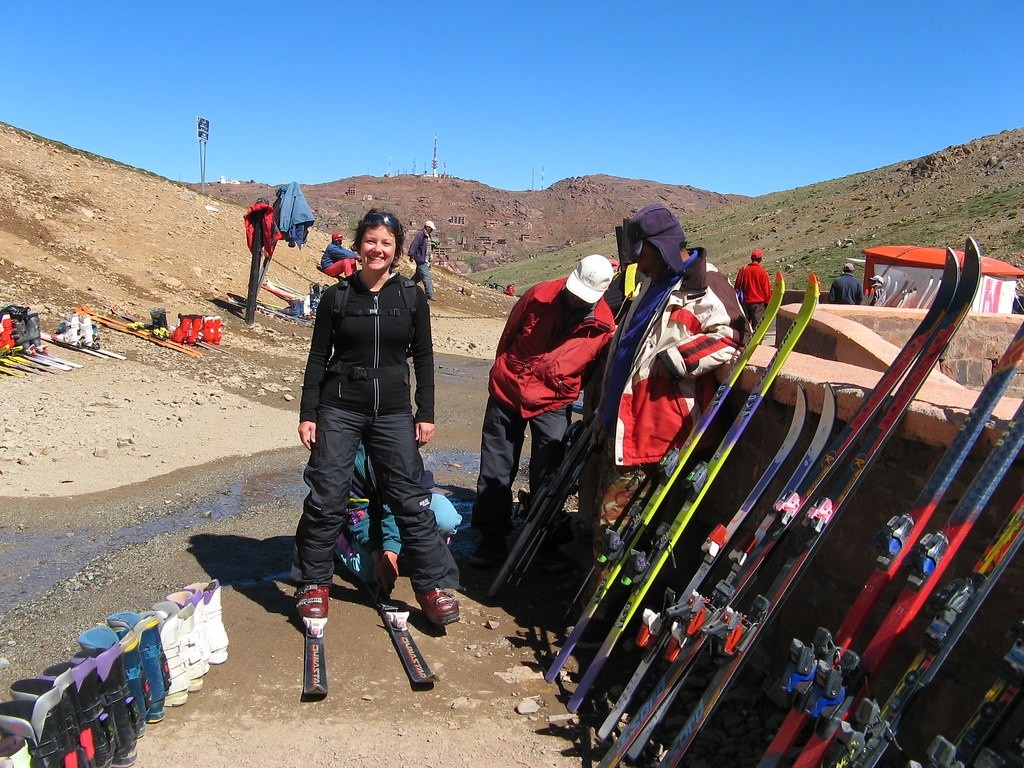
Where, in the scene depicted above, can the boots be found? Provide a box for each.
[0,308,35,356]
[54,313,94,346]
[27,313,48,354]
[148,308,221,346]
[0,576,229,768]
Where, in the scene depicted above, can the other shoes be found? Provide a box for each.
[470,536,507,566]
[280,298,304,318]
[430,297,436,301]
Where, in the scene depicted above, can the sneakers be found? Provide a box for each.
[296,581,329,618]
[416,587,460,626]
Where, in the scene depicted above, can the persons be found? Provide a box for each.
[334,434,463,595]
[865,275,887,306]
[482,273,497,289]
[321,231,359,281]
[408,220,440,303]
[298,209,461,626]
[827,262,863,305]
[733,248,773,345]
[819,294,825,304]
[568,203,753,650]
[470,255,617,567]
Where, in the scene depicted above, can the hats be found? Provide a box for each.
[626,203,686,274]
[751,249,762,260]
[845,262,855,272]
[332,232,345,241]
[871,275,883,284]
[566,253,614,304]
[425,221,437,230]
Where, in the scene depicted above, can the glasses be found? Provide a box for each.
[364,213,399,229]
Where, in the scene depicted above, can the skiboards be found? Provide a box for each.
[298,576,439,697]
[226,276,318,329]
[0,352,85,381]
[39,331,128,360]
[596,233,984,768]
[486,408,602,602]
[593,380,841,761]
[542,270,822,716]
[72,305,237,360]
[757,320,1024,768]
